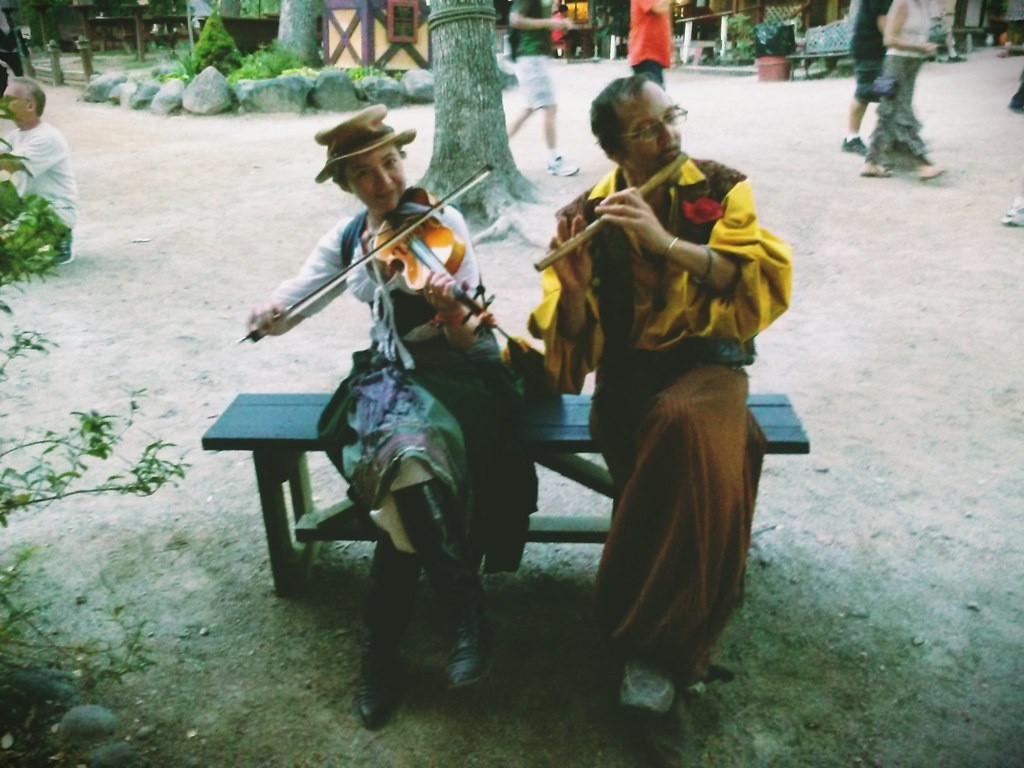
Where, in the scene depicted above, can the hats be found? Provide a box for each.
[314,103,415,185]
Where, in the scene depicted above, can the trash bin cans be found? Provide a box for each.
[755,24,796,58]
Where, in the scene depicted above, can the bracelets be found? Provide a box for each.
[665,236,679,259]
[434,309,465,326]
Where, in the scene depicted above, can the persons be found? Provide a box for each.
[507,0,580,176]
[553,4,574,59]
[0,77,78,264]
[843,1,966,180]
[628,0,672,87]
[248,104,544,726]
[592,9,615,48]
[530,77,791,716]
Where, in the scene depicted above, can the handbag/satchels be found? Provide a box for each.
[872,76,901,100]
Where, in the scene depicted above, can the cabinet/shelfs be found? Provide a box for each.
[81,14,192,52]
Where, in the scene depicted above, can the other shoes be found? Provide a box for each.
[53,230,74,265]
[547,157,580,178]
[620,657,677,716]
[862,167,894,176]
[919,165,947,184]
[645,703,691,768]
[842,138,868,156]
[1003,206,1024,227]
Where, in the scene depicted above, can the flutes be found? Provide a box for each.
[533,153,689,272]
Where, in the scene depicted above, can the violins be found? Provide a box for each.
[360,188,531,356]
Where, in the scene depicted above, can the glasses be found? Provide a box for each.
[618,106,689,143]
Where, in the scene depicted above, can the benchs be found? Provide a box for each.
[201,393,811,596]
[785,19,854,81]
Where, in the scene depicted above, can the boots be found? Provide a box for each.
[392,477,496,690]
[357,531,422,726]
